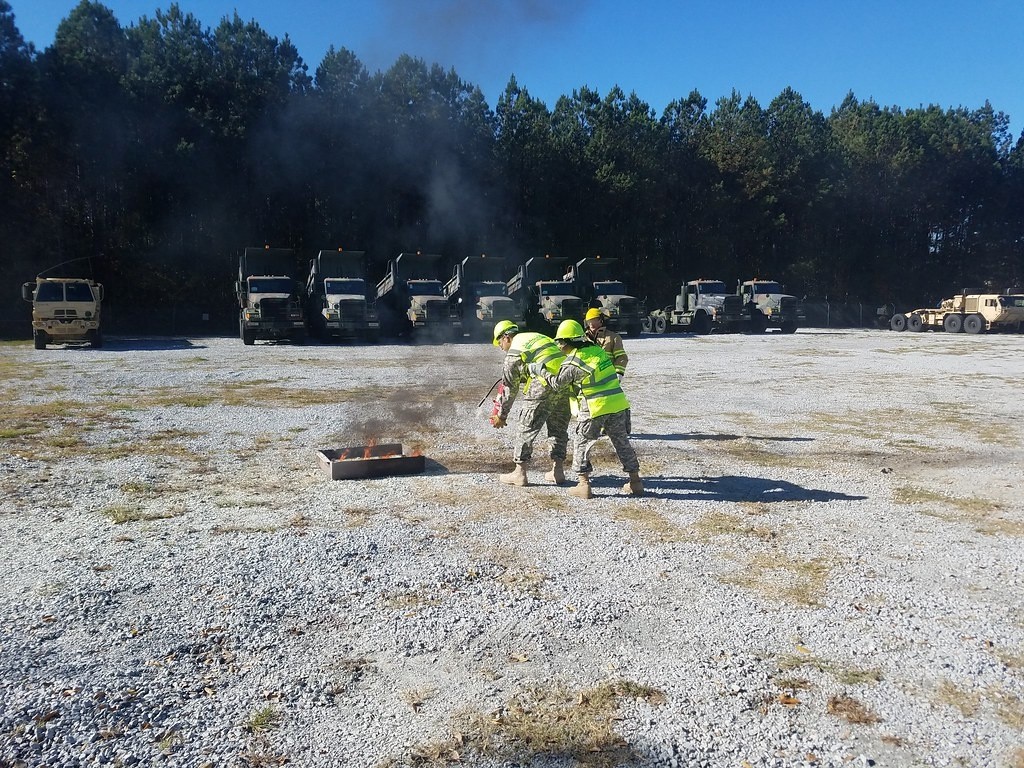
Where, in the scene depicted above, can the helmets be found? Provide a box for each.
[492,320,518,347]
[554,319,586,341]
[583,308,602,322]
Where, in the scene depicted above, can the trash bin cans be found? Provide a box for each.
[477,376,506,425]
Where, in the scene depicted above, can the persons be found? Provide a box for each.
[493,321,570,485]
[540,320,643,498]
[583,308,632,440]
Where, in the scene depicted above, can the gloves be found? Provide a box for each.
[490,415,507,429]
[527,362,546,376]
[616,373,623,383]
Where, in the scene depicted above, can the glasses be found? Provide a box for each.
[498,336,504,347]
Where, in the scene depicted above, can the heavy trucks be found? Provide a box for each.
[643,278,753,335]
[741,279,807,333]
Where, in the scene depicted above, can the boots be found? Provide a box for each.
[545,460,565,484]
[500,464,528,486]
[623,473,644,495]
[568,474,592,499]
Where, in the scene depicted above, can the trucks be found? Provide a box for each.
[22,275,104,350]
[232,245,641,339]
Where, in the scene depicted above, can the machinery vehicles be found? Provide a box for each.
[890,287,1024,334]
